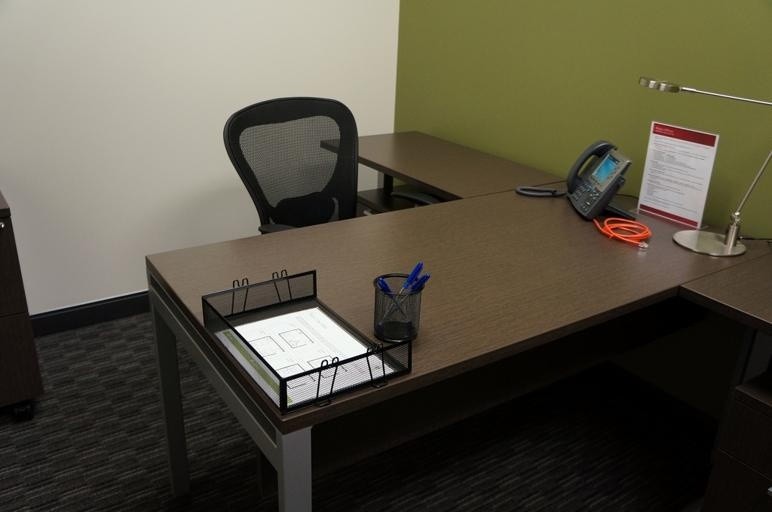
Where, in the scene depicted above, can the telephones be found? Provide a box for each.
[567,140,637,220]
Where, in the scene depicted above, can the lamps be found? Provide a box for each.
[638,75,772,256]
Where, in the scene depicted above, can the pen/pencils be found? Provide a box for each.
[378,261,430,331]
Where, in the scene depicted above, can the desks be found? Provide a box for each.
[146,130,772,510]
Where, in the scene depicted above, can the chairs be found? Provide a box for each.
[223,97,360,234]
